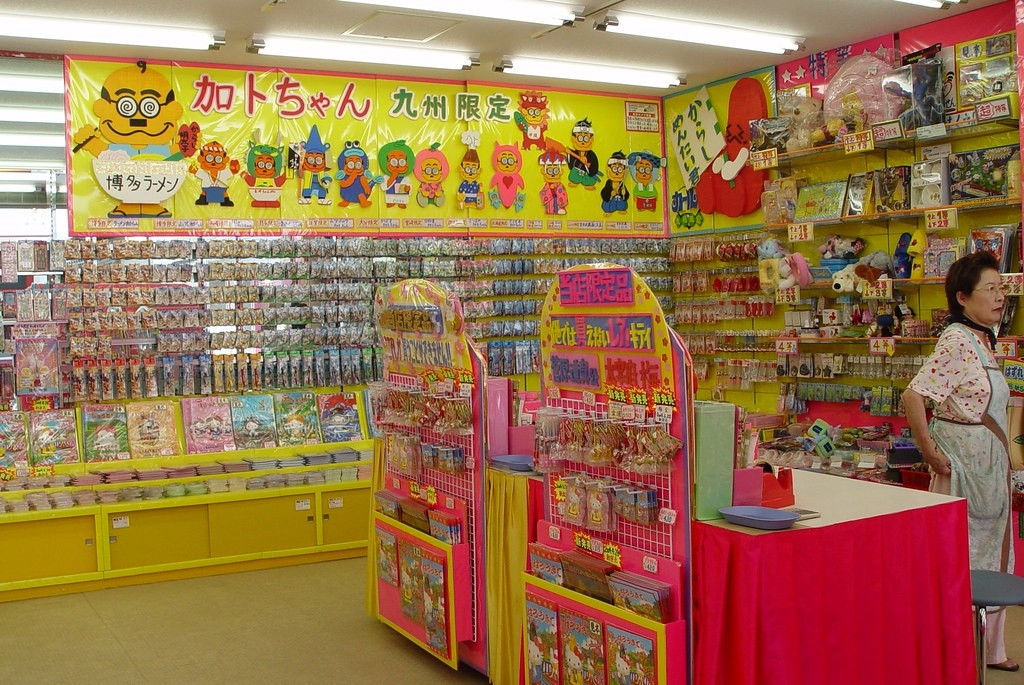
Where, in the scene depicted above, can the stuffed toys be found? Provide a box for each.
[757,235,927,297]
[786,86,872,153]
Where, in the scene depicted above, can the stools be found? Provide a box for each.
[969,571,1024,685]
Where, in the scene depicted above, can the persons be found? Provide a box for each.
[903,250,1019,671]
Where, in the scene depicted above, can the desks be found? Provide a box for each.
[686,463,979,684]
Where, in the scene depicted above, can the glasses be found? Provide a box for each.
[974,286,1010,296]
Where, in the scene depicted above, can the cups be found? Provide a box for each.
[914,163,930,177]
[1007,160,1021,203]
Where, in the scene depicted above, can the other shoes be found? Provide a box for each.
[987,660,1019,671]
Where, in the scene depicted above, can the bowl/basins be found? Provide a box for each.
[921,185,941,205]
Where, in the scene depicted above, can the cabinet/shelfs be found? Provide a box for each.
[0,475,376,603]
[750,114,1024,354]
